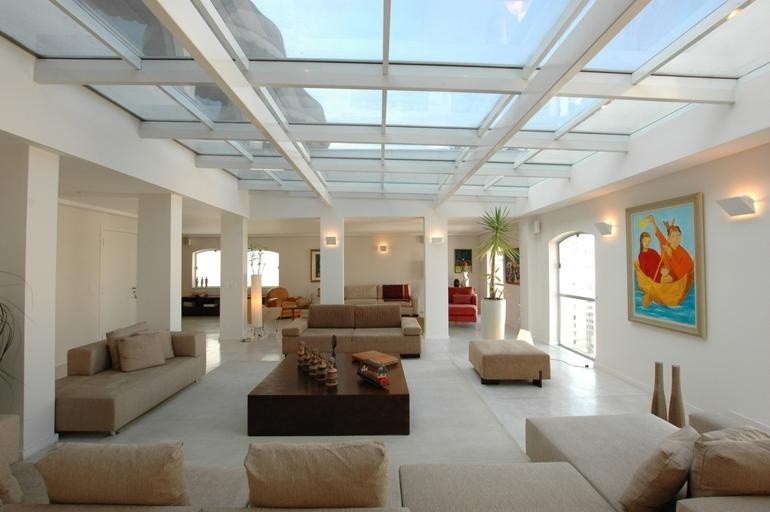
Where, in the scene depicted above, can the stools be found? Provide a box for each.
[468,338,551,389]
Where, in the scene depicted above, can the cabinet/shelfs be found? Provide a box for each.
[181,296,221,318]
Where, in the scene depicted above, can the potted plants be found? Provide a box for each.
[471,204,522,339]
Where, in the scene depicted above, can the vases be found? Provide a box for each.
[462,271,470,287]
[650,360,668,420]
[669,366,687,428]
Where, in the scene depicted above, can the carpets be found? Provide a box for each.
[100,357,532,466]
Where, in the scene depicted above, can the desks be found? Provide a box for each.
[288,305,310,321]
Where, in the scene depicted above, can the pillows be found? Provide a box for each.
[382,284,403,299]
[453,294,473,304]
[386,284,411,302]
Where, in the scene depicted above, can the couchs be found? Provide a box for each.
[310,284,419,317]
[53,321,207,438]
[0,437,618,511]
[523,404,769,511]
[281,303,422,360]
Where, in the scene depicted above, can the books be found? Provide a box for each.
[352,350,399,368]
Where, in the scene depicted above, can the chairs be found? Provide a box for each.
[247,296,283,334]
[448,286,478,324]
[264,286,303,320]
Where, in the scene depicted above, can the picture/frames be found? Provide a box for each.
[310,249,321,283]
[454,248,473,273]
[622,189,710,339]
[505,247,521,286]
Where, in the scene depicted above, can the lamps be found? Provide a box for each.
[595,221,612,235]
[717,195,757,217]
[250,273,263,338]
